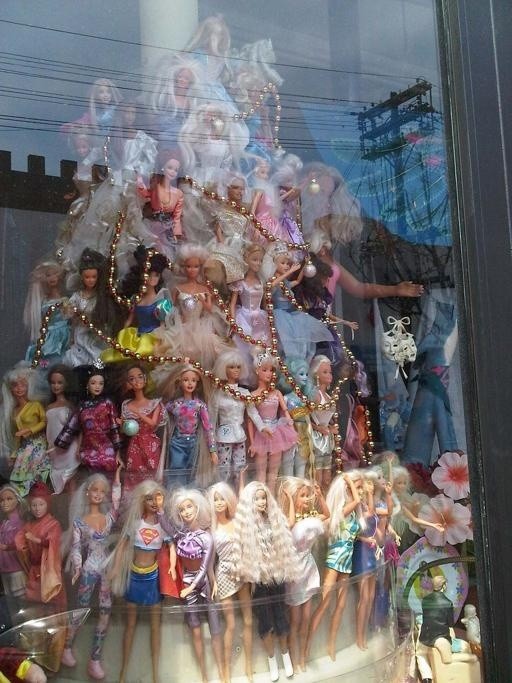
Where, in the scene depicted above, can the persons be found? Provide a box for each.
[1,10,423,498]
[0,11,483,683]
[1,468,455,683]
